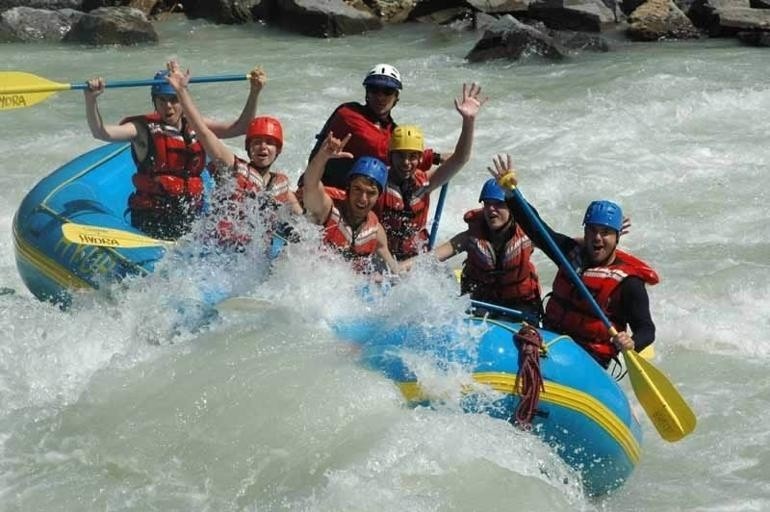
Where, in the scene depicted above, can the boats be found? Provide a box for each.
[13,130,647,512]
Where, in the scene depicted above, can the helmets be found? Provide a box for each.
[152,72,178,95]
[362,63,401,101]
[247,116,283,147]
[581,201,624,234]
[347,156,389,193]
[389,124,425,154]
[478,179,509,205]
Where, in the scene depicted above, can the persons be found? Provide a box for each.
[77,62,264,240]
[372,81,487,264]
[482,152,660,373]
[159,61,308,257]
[365,177,635,328]
[302,128,400,310]
[292,61,455,216]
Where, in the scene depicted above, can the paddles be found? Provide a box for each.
[496,168,696,443]
[0,71,259,110]
[61,223,182,249]
